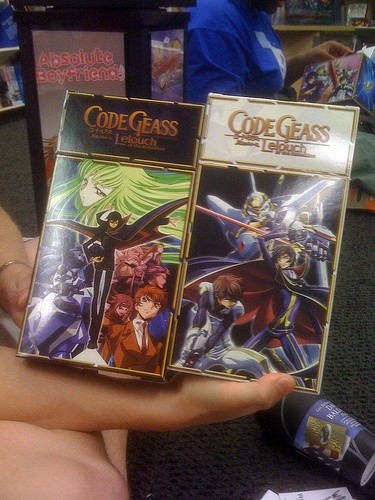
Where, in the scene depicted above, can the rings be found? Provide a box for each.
[0,260,30,274]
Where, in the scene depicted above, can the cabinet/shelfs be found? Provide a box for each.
[272,0,375,59]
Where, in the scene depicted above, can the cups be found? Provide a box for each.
[272,387,375,486]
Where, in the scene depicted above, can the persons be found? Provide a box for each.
[179,1,374,197]
[0,205,295,500]
